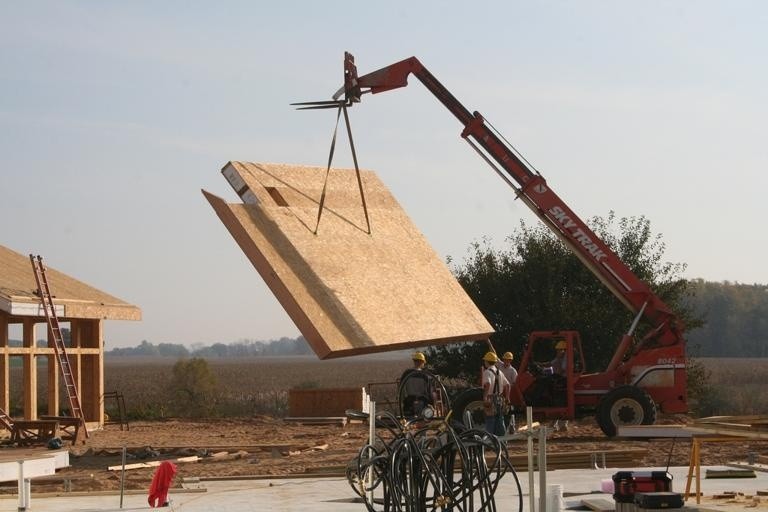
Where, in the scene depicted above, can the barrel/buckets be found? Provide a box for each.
[546,484,563,512]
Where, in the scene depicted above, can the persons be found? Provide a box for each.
[486,338,520,434]
[548,418,568,435]
[525,340,568,407]
[411,351,426,370]
[481,351,510,451]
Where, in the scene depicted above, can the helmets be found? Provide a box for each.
[554,340,568,350]
[483,352,497,362]
[503,352,513,359]
[412,352,426,363]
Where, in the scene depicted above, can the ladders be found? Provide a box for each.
[0,408,33,447]
[29,253,91,442]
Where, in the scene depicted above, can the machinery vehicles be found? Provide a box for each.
[289,51,689,438]
